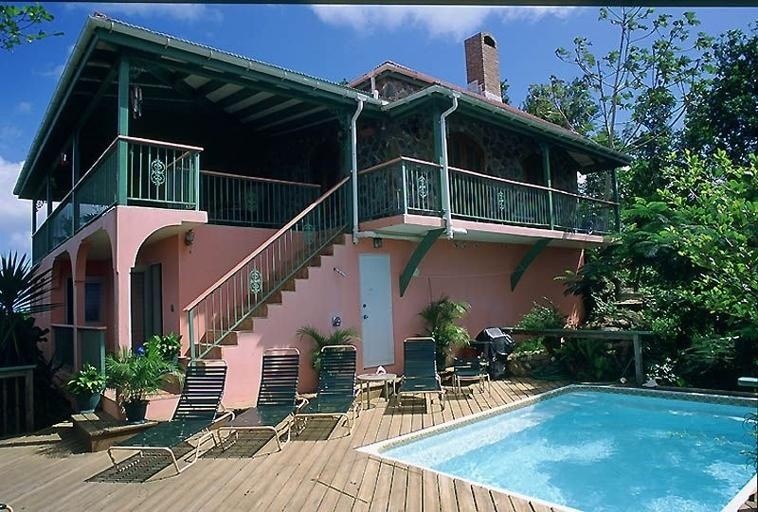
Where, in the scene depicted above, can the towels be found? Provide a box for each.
[475,327,508,381]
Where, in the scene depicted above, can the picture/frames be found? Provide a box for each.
[358,370,394,411]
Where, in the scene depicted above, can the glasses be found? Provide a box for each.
[129,82,144,118]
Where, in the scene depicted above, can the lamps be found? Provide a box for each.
[107,359,235,476]
[396,332,446,412]
[452,355,492,399]
[296,342,363,446]
[218,343,301,456]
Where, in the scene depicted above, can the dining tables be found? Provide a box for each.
[66,332,185,423]
